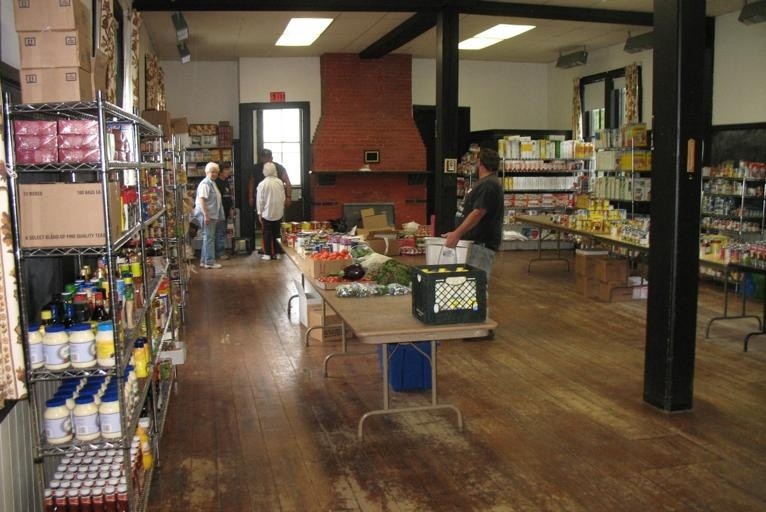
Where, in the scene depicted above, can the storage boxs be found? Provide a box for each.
[575,251,648,302]
[376,339,441,392]
[423,236,473,268]
[307,303,352,341]
[292,278,323,328]
[12,0,112,107]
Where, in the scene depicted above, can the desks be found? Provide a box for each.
[515,207,766,352]
[276,233,499,442]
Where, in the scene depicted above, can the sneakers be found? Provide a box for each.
[200,262,205,267]
[205,263,222,269]
[463,330,494,340]
[262,254,271,260]
[276,254,282,259]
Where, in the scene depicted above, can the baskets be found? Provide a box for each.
[411,263,487,324]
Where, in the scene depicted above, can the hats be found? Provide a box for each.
[261,149,272,156]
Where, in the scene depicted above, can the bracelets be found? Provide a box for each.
[286,196,291,198]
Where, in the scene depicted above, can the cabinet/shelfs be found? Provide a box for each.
[458,131,590,252]
[697,163,765,292]
[586,136,651,269]
[184,141,236,255]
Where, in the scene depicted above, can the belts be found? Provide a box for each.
[477,242,497,251]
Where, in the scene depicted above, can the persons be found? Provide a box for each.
[255,162,286,261]
[248,149,292,255]
[441,148,505,341]
[194,162,226,269]
[215,161,234,260]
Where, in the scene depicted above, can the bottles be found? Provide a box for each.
[139,167,165,231]
[36,404,163,511]
[26,227,171,377]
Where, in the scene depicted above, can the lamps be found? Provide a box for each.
[623,29,656,56]
[171,10,192,64]
[556,44,589,70]
[736,0,765,27]
